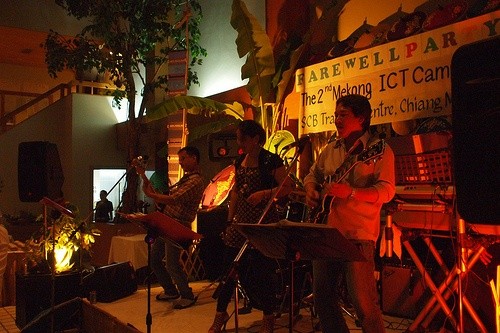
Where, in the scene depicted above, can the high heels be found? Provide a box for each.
[262,314,273,333]
[209,310,229,333]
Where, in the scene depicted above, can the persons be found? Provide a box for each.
[95,191,114,223]
[208,119,296,333]
[302,94,395,333]
[142,145,206,309]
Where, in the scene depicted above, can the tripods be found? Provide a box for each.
[191,152,362,333]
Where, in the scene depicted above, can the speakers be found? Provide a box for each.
[378,265,447,320]
[449,35,500,224]
[20,298,141,333]
[18,140,64,202]
[12,272,82,330]
[82,261,137,303]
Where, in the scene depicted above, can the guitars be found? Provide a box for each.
[131,154,168,213]
[306,136,387,225]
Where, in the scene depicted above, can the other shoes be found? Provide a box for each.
[156,292,179,300]
[176,293,193,308]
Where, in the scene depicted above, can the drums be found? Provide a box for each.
[237,247,306,314]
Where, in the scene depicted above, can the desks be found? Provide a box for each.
[92,222,148,272]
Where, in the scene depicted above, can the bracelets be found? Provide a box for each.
[263,190,267,200]
[226,220,233,226]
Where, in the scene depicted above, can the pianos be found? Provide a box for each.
[393,201,500,333]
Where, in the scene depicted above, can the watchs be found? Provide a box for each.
[348,186,357,201]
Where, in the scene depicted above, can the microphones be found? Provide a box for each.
[282,133,314,150]
